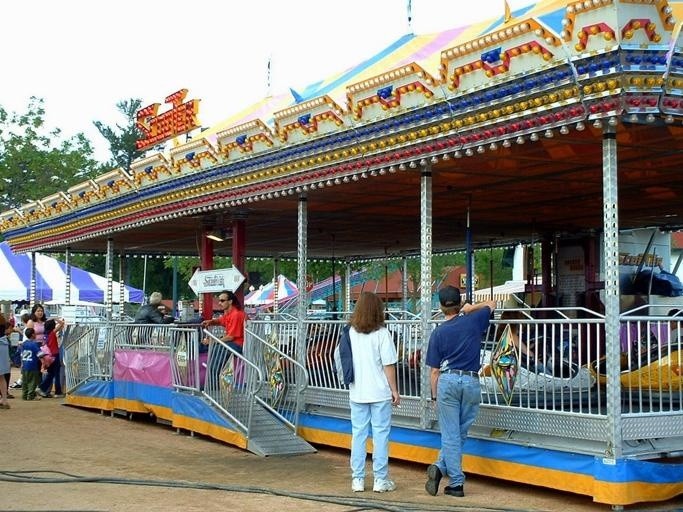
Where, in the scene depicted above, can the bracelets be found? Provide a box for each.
[431,397,436,401]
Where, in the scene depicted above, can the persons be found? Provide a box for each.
[496,310,551,376]
[424,285,497,498]
[133,291,175,344]
[200,289,250,392]
[666,309,683,345]
[630,319,658,369]
[333,291,400,493]
[0,301,64,410]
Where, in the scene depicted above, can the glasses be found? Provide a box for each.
[218,298,229,303]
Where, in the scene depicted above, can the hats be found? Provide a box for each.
[437,284,462,307]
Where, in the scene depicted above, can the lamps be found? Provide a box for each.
[205,228,233,243]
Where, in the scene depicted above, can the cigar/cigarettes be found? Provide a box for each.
[396,405,402,409]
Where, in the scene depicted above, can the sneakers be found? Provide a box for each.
[53,393,65,398]
[423,463,443,496]
[33,383,52,399]
[6,394,14,399]
[351,477,365,494]
[442,484,465,497]
[21,395,41,401]
[0,401,12,410]
[8,381,22,388]
[371,476,398,493]
